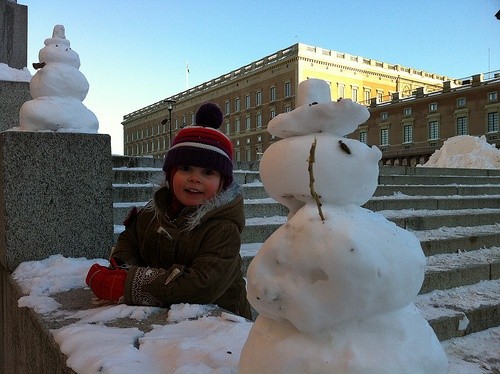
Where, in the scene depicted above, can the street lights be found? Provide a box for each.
[164,97,176,148]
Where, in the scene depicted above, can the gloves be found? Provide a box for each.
[86,263,127,300]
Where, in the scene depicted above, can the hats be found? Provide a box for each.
[163,103,234,182]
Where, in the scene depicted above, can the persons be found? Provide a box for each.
[85,102,253,322]
